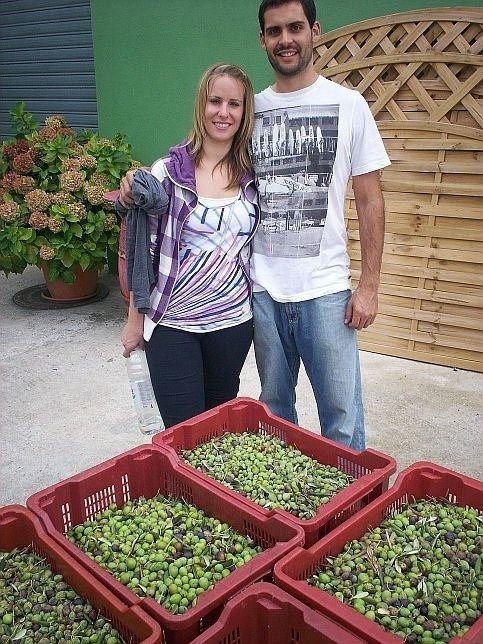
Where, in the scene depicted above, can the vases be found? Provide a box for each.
[40,262,98,302]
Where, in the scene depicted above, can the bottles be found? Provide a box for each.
[125,347,164,435]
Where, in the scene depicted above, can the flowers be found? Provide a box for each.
[0,102,139,284]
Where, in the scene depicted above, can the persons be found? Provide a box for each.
[118,0,393,451]
[121,63,262,429]
